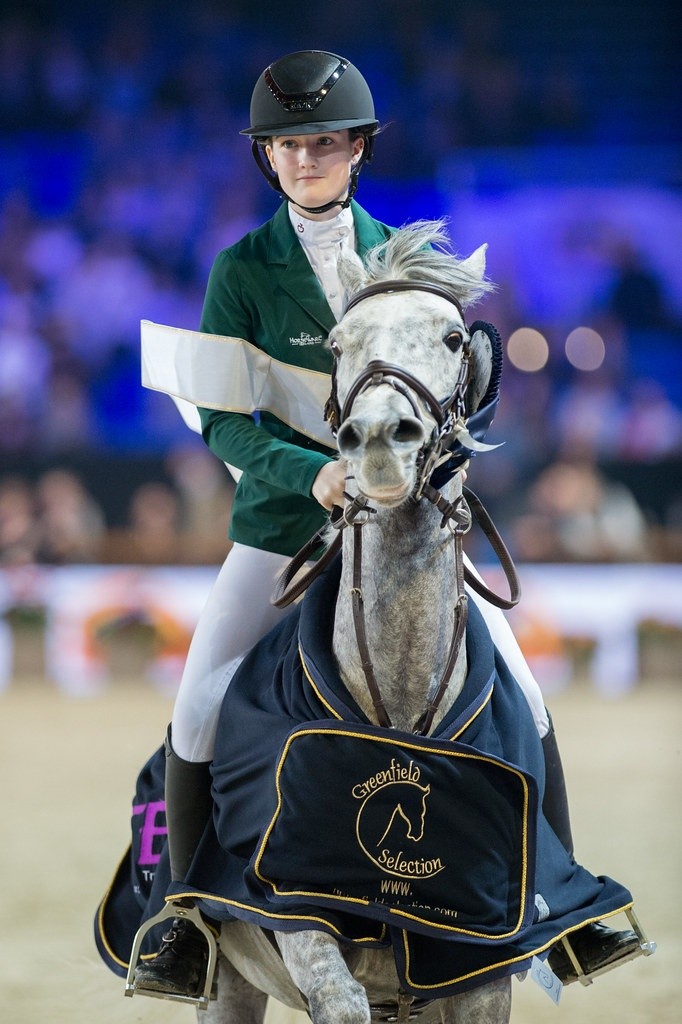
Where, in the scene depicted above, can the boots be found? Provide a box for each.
[540,706,638,987]
[137,722,219,997]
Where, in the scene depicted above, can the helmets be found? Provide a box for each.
[238,49,378,137]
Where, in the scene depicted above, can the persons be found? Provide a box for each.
[130,51,639,1003]
[0,440,653,675]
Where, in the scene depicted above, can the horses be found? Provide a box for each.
[194,215,546,1024]
